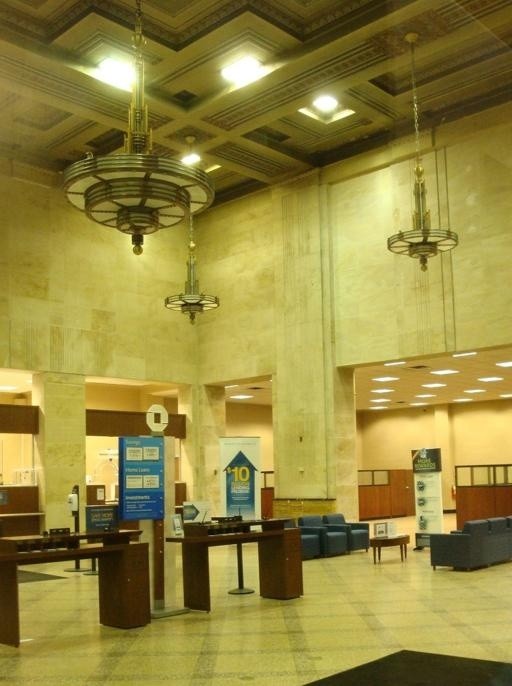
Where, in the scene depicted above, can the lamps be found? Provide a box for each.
[165,136,222,324]
[63,2,212,257]
[384,29,462,274]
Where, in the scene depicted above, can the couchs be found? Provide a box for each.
[429,513,511,575]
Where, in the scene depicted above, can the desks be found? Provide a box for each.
[0,526,153,649]
[164,517,307,612]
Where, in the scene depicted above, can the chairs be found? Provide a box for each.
[279,512,370,562]
